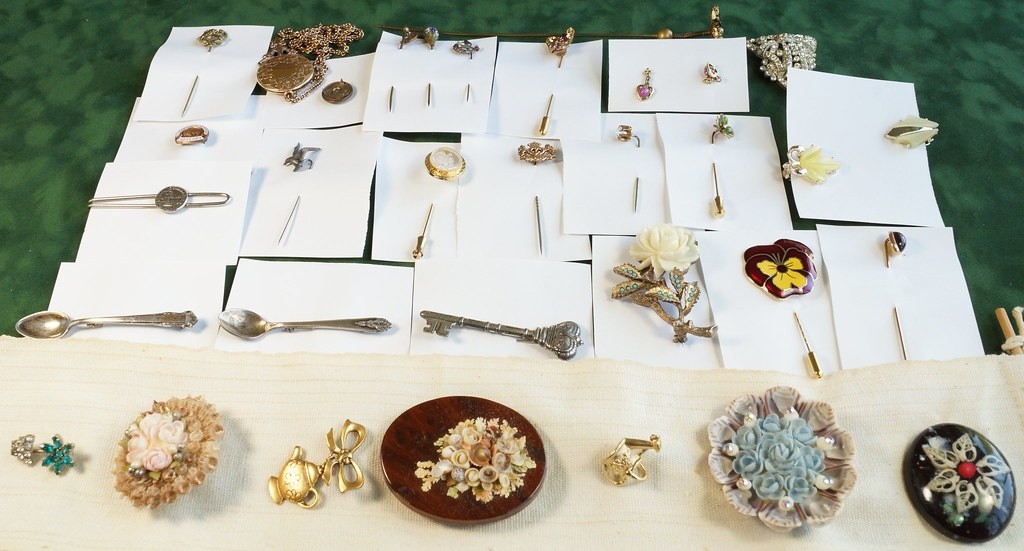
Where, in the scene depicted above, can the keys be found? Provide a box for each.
[420,311,583,360]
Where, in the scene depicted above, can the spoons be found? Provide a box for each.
[14,310,197,341]
[218,308,392,341]
